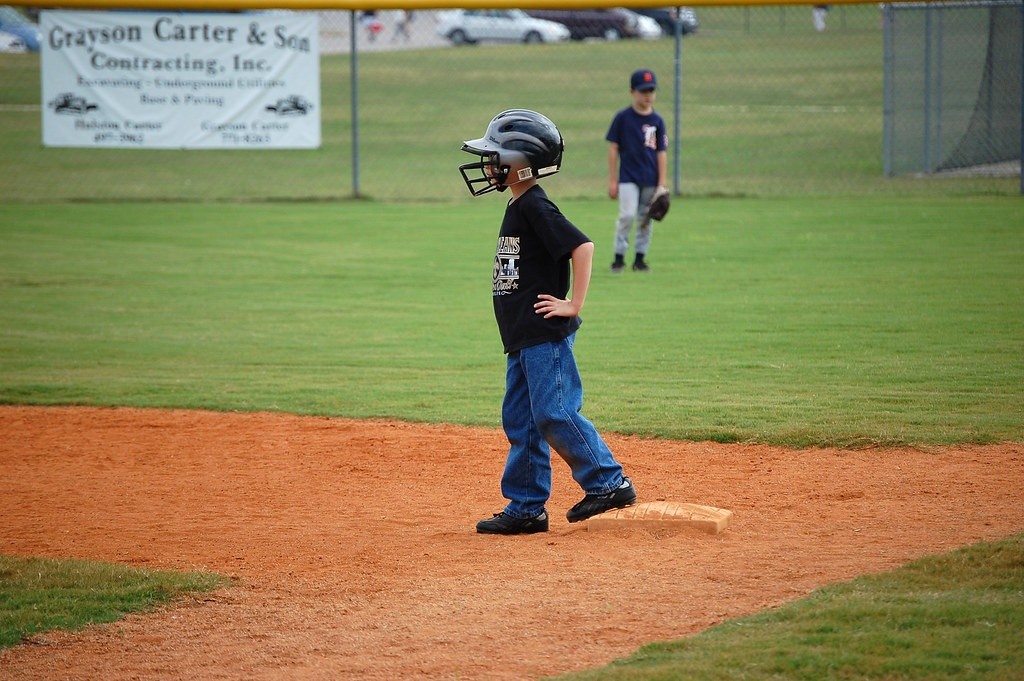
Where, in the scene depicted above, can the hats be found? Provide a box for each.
[631,70,657,91]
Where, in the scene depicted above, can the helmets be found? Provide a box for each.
[459,109,564,197]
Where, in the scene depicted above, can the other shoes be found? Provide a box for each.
[633,262,652,273]
[611,262,626,275]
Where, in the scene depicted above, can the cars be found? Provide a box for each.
[437,6,570,44]
[525,7,635,40]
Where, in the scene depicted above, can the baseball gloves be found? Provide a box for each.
[640,185,671,230]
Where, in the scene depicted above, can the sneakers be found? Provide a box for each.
[565,477,637,523]
[476,508,549,535]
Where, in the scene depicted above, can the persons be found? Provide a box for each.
[460,109,636,535]
[812,4,830,32]
[606,70,668,273]
